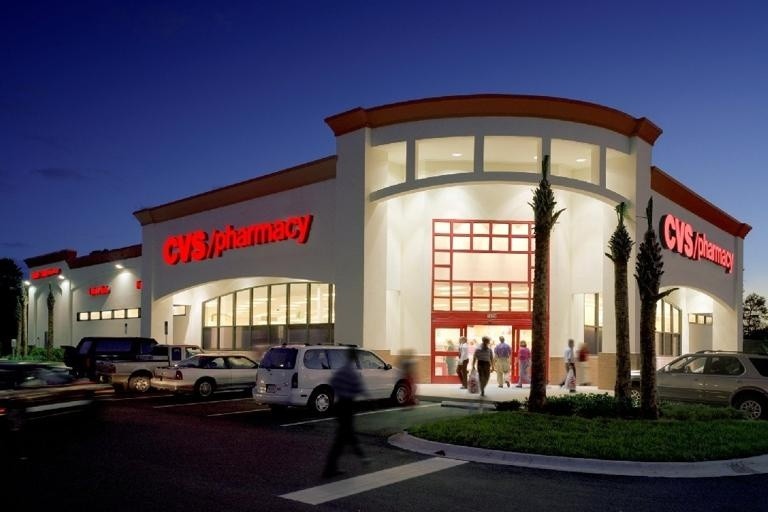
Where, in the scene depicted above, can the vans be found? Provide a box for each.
[249,341,418,419]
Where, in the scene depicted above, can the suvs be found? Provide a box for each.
[628,347,768,421]
[62,336,160,384]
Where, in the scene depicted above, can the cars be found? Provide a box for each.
[1,361,101,469]
[151,352,259,398]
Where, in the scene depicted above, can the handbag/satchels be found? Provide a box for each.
[466,372,480,395]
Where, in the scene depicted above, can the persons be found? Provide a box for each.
[319,348,369,480]
[445,336,531,397]
[576,342,592,387]
[557,338,578,393]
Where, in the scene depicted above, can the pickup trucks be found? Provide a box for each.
[94,343,207,395]
